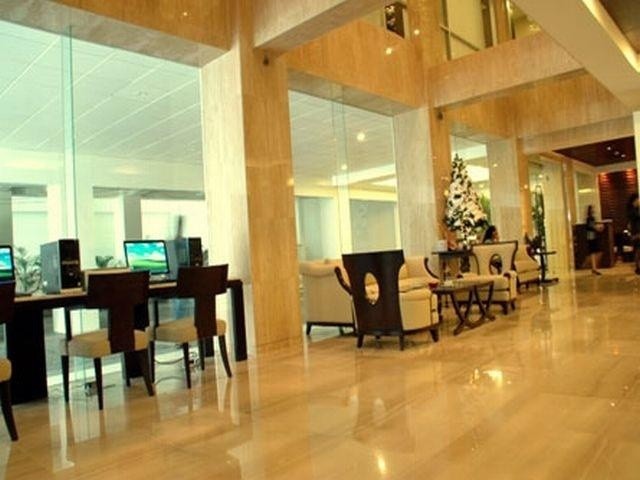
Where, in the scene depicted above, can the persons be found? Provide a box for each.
[627,193,640,276]
[482,225,499,244]
[582,204,604,275]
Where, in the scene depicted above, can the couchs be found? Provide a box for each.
[298,256,441,334]
[427,243,539,306]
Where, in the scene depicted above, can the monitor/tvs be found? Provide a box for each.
[123,239,170,275]
[0,245,15,279]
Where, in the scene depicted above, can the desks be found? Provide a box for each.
[0,275,248,406]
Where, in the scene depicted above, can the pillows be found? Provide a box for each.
[324,256,424,284]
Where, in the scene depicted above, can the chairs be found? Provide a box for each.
[145,264,232,389]
[58,269,156,411]
[455,241,519,316]
[0,281,19,441]
[341,249,441,349]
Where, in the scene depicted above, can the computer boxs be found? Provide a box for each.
[38,238,82,293]
[172,237,203,279]
